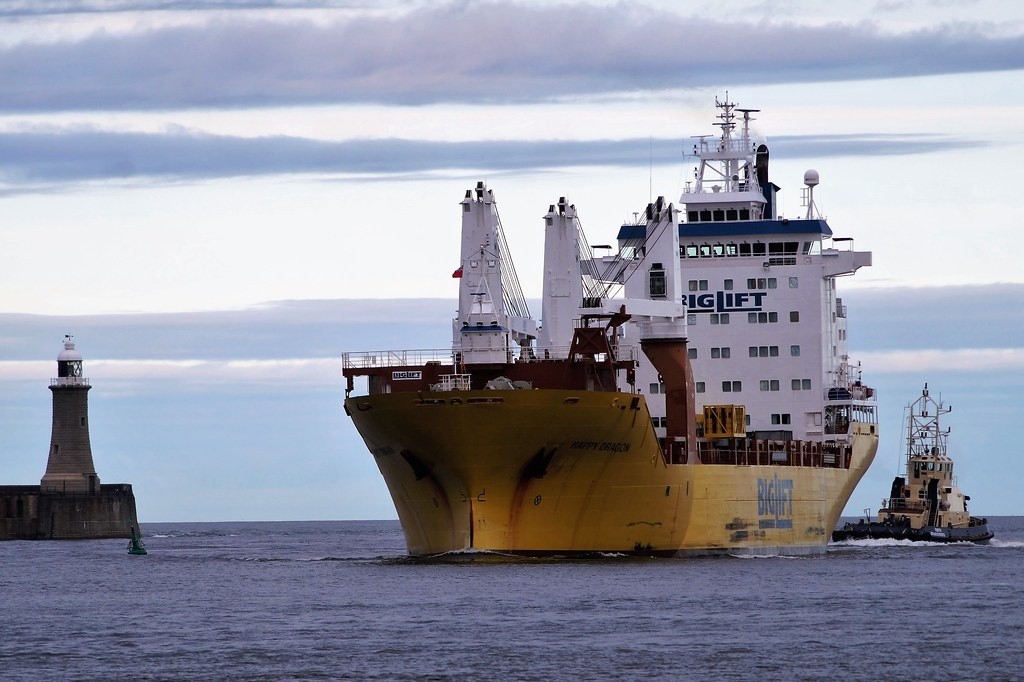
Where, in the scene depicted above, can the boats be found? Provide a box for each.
[830,382,994,548]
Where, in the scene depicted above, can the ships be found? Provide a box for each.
[339,92,882,553]
